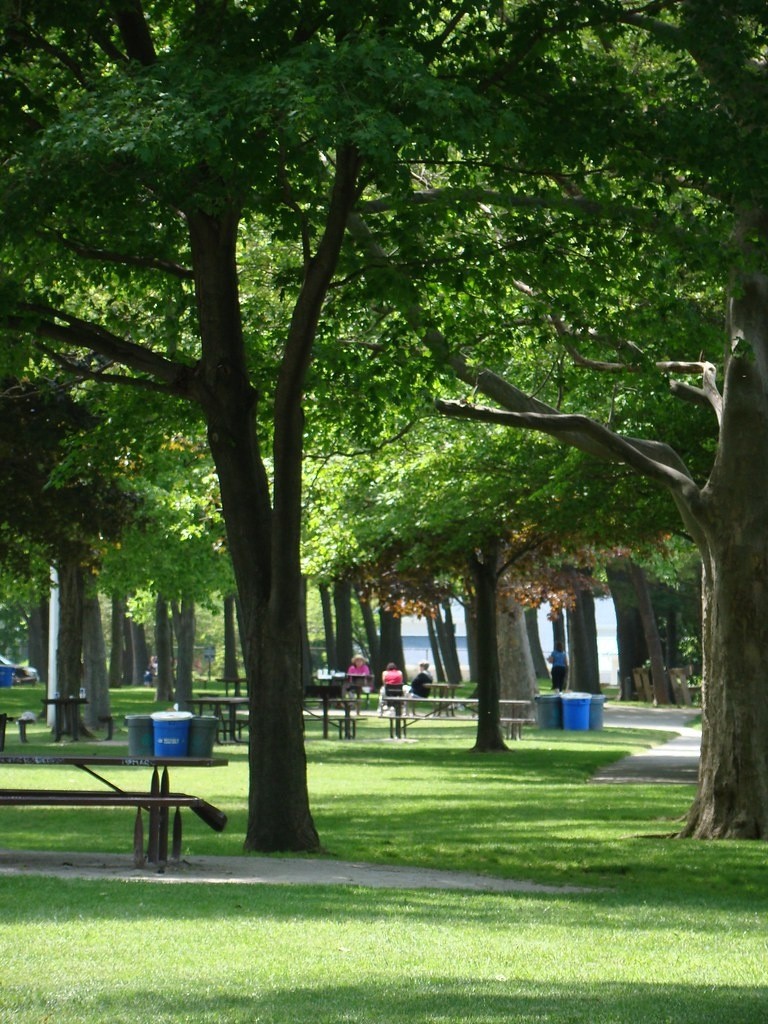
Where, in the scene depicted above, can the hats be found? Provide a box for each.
[350,654,367,663]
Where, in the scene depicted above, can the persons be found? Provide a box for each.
[346,655,433,716]
[547,643,569,693]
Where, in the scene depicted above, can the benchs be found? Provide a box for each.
[380,714,536,741]
[0,789,204,865]
[18,719,33,743]
[97,714,115,741]
[217,717,370,741]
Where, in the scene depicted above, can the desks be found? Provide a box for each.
[0,754,229,867]
[384,698,531,738]
[40,697,89,742]
[186,697,365,739]
[216,678,247,697]
[425,683,465,717]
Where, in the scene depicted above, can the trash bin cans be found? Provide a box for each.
[0,665,13,688]
[534,694,560,729]
[150,711,193,757]
[188,716,220,758]
[589,695,607,731]
[124,714,154,756]
[560,692,592,731]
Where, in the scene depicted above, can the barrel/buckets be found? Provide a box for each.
[188,715,219,758]
[590,693,607,730]
[152,711,194,757]
[0,666,15,688]
[560,692,592,731]
[124,715,153,756]
[534,695,561,729]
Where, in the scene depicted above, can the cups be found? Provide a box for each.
[323,669,328,675]
[317,670,322,676]
[330,670,335,675]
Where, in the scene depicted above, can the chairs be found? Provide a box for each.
[385,683,404,708]
